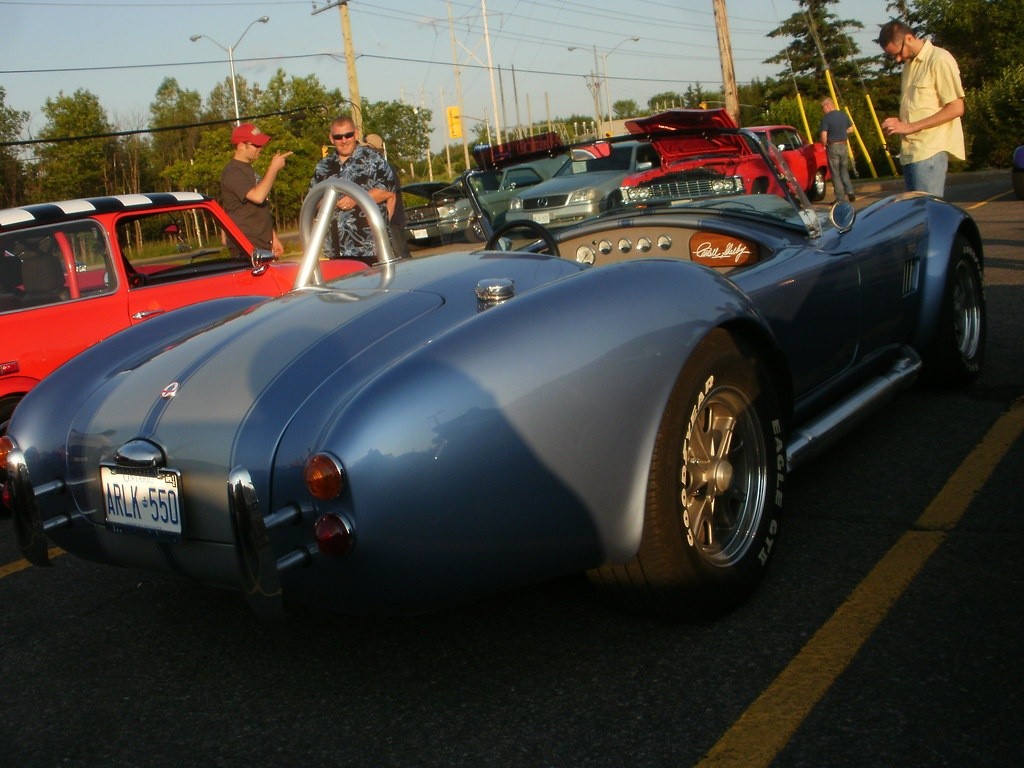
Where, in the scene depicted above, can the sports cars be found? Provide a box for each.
[0,179,991,625]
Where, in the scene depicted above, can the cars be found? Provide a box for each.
[402,164,548,245]
[506,140,661,238]
[0,190,372,510]
[620,108,833,209]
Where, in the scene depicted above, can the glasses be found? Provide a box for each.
[820,105,827,110]
[332,131,356,140]
[247,144,260,148]
[891,40,907,58]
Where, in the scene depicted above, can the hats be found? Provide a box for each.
[358,133,384,149]
[231,124,271,146]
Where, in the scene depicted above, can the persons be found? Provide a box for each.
[880,20,965,197]
[220,123,293,261]
[308,115,411,266]
[821,98,855,205]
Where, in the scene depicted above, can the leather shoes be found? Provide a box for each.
[848,194,857,203]
[829,198,844,206]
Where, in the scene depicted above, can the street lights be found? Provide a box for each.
[567,36,641,133]
[190,15,271,127]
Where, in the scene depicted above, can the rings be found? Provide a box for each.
[891,131,894,135]
[891,127,892,130]
